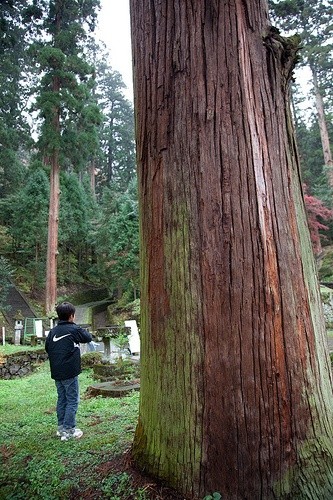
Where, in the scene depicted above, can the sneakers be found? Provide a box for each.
[57,428,84,442]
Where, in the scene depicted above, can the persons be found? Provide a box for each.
[45,301,94,441]
